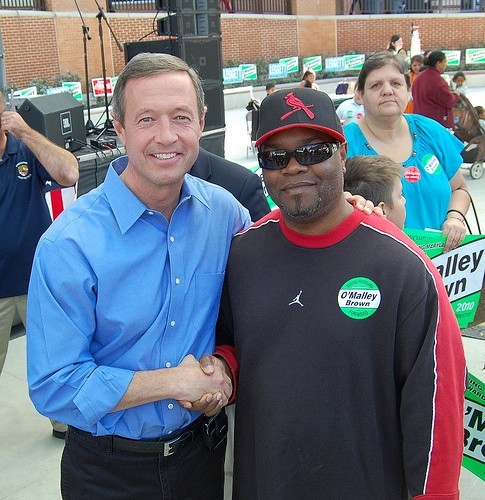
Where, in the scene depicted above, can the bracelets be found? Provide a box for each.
[446,216,465,225]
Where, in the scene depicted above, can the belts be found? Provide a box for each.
[66,407,226,457]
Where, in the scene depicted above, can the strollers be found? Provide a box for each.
[452,91,485,180]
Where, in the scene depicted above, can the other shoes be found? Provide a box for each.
[52,429,68,438]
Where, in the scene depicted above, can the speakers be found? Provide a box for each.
[17,92,87,152]
[123,1,226,159]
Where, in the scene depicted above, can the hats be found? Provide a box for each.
[254,87,348,148]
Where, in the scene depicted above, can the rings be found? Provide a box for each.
[4,121,5,124]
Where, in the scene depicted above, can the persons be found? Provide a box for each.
[296,70,320,90]
[26,51,382,500]
[265,83,276,96]
[192,147,271,222]
[340,51,469,254]
[0,90,80,440]
[341,155,407,229]
[387,34,485,134]
[181,89,465,500]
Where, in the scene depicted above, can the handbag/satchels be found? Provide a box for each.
[446,187,485,330]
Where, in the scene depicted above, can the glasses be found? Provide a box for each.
[256,140,340,170]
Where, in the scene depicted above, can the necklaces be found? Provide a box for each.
[363,122,402,143]
